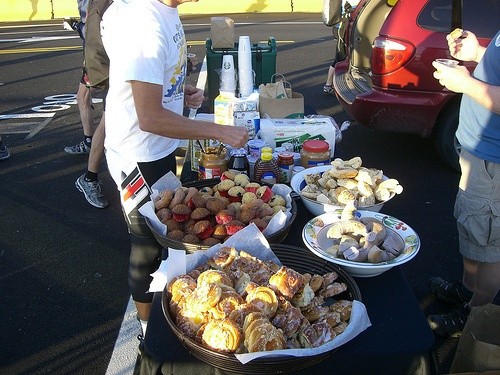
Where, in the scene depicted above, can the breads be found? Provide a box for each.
[325,200,402,263]
[450,28,463,39]
[303,157,402,206]
[166,246,351,354]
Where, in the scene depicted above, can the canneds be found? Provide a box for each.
[300,140,331,168]
[198,146,230,179]
[276,153,294,184]
[291,166,305,178]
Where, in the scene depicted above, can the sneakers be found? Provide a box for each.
[430,277,469,312]
[427,302,472,338]
[323,84,336,93]
[74,173,109,208]
[0,145,11,160]
[63,141,90,155]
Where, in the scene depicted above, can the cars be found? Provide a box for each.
[322,0,500,172]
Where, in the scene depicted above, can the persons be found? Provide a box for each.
[322,0,361,93]
[0,136,9,160]
[63,0,133,209]
[427,28,500,338]
[104,0,251,355]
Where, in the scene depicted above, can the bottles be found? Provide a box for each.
[198,146,229,179]
[228,153,251,180]
[248,139,305,188]
[299,140,331,168]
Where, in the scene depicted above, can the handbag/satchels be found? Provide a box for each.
[449,304,500,373]
[259,73,305,119]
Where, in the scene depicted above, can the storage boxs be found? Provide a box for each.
[260,119,336,157]
[209,18,235,49]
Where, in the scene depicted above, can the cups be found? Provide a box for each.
[436,58,459,86]
[221,54,236,92]
[238,36,253,97]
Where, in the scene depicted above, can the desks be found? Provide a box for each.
[135,123,436,375]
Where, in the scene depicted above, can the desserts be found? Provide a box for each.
[155,170,286,246]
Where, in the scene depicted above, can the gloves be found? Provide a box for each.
[64,17,78,31]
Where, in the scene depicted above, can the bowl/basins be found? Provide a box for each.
[144,179,302,252]
[162,243,361,375]
[290,165,390,217]
[301,210,421,277]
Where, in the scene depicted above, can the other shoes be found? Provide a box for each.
[137,332,145,351]
